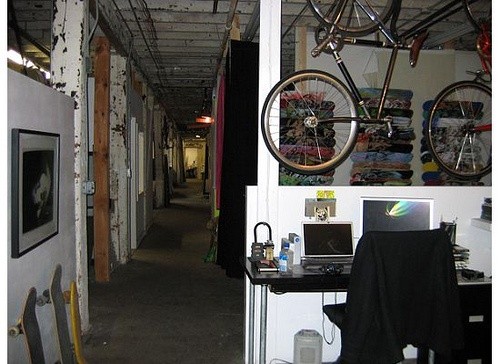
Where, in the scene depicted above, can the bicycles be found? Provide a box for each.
[260,0,492,180]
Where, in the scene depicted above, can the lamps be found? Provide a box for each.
[199,88,213,119]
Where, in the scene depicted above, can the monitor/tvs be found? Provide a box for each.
[359,197,433,236]
[302,221,354,258]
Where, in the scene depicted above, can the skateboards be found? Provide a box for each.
[8,286,45,364]
[36,263,75,364]
[63,281,87,364]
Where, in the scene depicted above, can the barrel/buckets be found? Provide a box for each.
[294,329,322,364]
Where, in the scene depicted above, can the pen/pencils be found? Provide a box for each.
[445,216,458,242]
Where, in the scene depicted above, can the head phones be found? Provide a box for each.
[325,263,345,274]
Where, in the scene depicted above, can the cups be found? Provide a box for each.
[441,222,455,246]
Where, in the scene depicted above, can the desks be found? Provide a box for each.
[242,256,492,364]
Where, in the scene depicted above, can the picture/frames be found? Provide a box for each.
[11,126,62,258]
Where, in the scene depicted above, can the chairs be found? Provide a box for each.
[322,231,470,364]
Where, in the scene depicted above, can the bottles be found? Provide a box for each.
[279,244,293,276]
